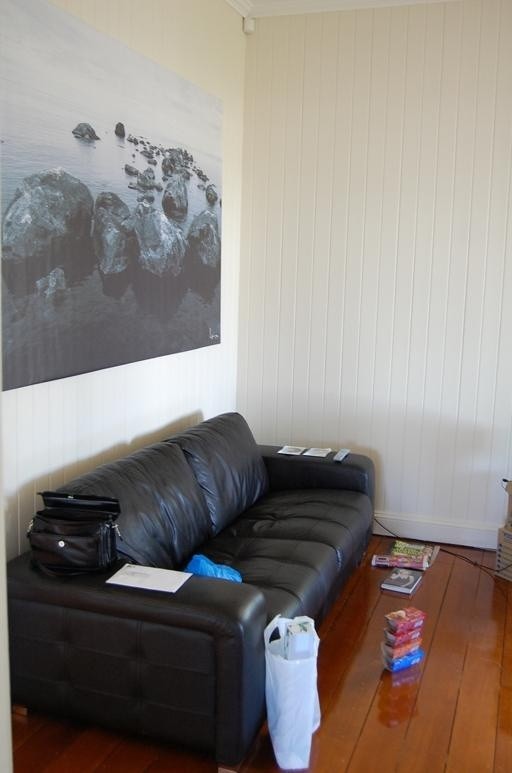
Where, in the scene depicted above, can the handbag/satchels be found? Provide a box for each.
[25,490,123,580]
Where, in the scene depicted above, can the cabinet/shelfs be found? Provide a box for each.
[495,478,512,582]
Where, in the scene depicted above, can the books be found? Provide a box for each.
[372,540,441,595]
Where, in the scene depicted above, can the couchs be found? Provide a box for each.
[6,414,373,766]
